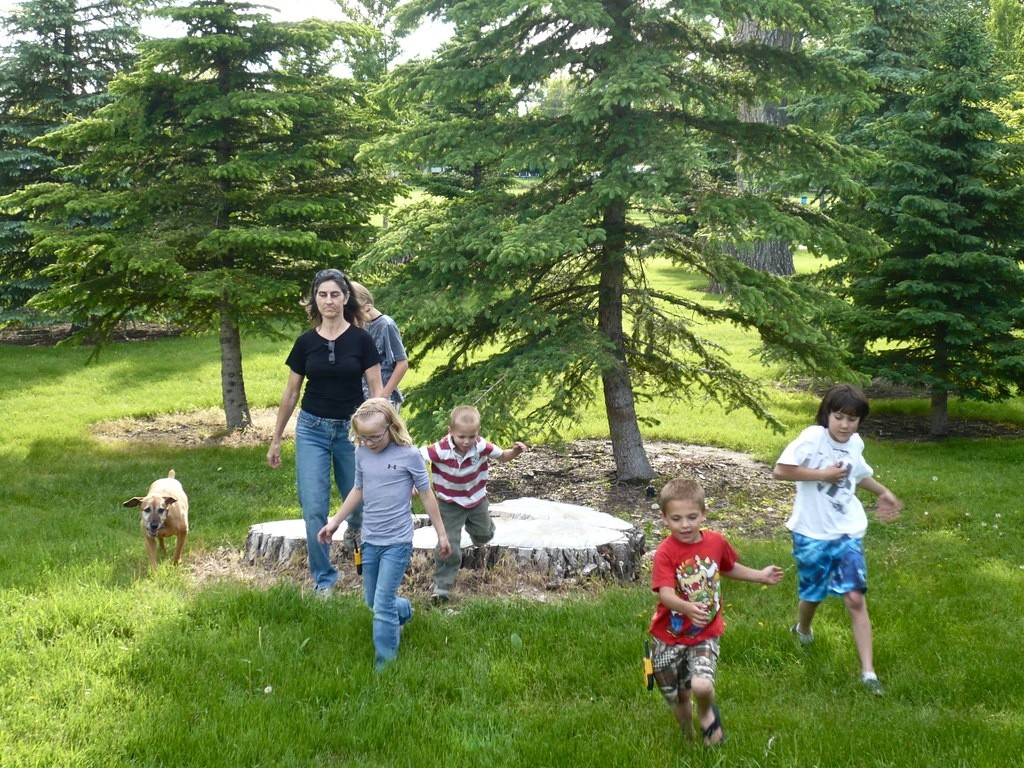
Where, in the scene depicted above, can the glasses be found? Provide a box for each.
[315,269,349,287]
[357,424,391,442]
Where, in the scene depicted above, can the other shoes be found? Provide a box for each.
[316,583,335,599]
[430,588,449,606]
[790,624,814,646]
[398,614,412,629]
[865,678,884,697]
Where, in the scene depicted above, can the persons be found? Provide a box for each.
[413,404,527,603]
[266,268,384,600]
[648,475,784,749]
[773,383,907,696]
[349,279,409,412]
[317,397,452,671]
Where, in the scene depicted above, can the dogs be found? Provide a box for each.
[123,469,190,571]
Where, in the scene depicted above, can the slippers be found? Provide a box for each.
[700,704,723,747]
